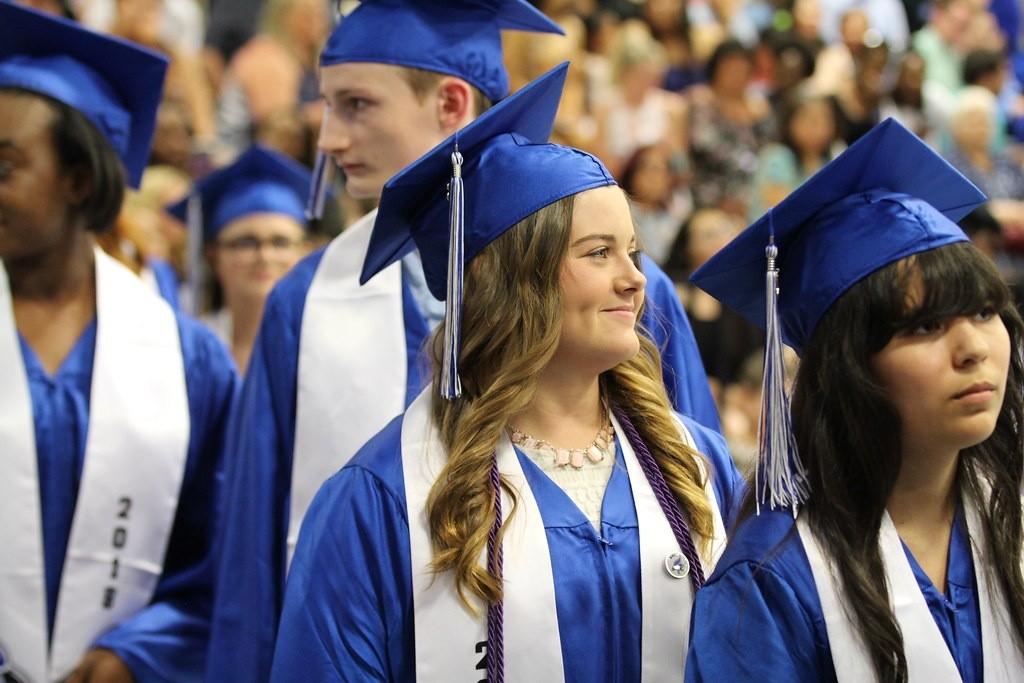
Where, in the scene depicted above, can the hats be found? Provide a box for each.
[1,0,169,192]
[357,59,615,403]
[165,144,337,314]
[305,0,566,220]
[687,118,989,521]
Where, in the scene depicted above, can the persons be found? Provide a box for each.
[210,0,722,683]
[269,61,745,683]
[3,0,1024,480]
[682,118,1023,683]
[0,0,237,683]
[168,144,332,368]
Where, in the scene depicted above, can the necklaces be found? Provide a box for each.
[509,407,614,469]
